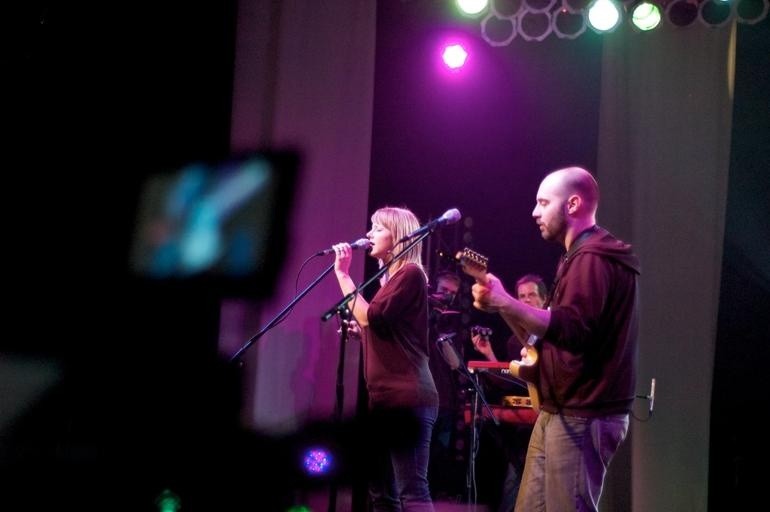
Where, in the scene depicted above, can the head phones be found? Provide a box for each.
[428,271,462,304]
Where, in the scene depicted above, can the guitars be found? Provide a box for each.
[455,248,543,413]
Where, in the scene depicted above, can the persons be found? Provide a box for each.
[473,274,550,396]
[333,204,439,511]
[472,164,641,512]
[429,265,468,500]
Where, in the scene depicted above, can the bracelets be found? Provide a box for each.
[437,335,451,351]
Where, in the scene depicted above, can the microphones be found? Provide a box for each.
[397,207,462,244]
[316,238,370,257]
